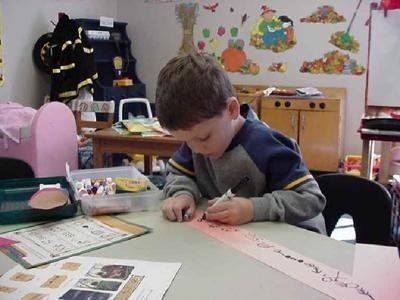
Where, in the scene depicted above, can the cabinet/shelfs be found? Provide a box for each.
[232,83,260,122]
[75,18,148,124]
[260,83,347,173]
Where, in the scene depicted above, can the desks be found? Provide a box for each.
[84,115,189,177]
[358,116,399,182]
[1,197,399,300]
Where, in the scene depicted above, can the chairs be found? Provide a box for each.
[71,98,116,167]
[118,96,165,174]
[0,157,36,182]
[312,174,394,246]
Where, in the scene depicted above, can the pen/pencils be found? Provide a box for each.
[201,176,250,220]
[73,177,130,213]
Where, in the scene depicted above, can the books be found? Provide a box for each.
[111,116,175,139]
[295,86,322,97]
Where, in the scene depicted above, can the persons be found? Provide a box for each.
[155,51,327,238]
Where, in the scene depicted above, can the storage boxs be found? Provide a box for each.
[65,161,165,216]
[0,176,75,225]
